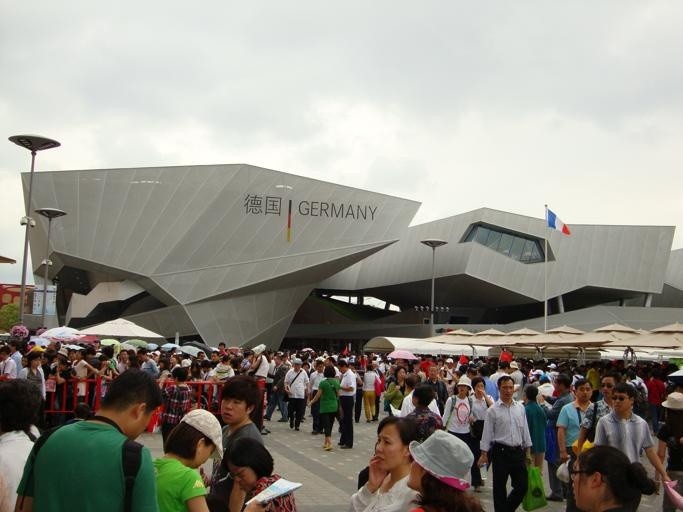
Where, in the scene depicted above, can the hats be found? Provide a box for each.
[179,408,223,459]
[661,392,683,410]
[547,364,556,369]
[409,429,475,491]
[510,361,518,368]
[293,358,303,365]
[456,375,473,390]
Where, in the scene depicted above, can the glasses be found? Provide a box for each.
[612,395,624,400]
[601,384,613,388]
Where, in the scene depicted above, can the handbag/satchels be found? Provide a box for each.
[523,464,547,510]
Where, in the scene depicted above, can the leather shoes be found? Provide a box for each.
[312,431,352,451]
[261,415,305,434]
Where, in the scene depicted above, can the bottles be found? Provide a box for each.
[479,462,488,480]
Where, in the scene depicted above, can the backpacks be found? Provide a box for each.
[630,382,650,411]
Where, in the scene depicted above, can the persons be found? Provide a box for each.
[2,340,683,512]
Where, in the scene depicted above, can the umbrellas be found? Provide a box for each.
[39,326,86,342]
[30,334,50,347]
[425,322,683,360]
[73,318,164,339]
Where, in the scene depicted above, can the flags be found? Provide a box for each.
[545,207,572,238]
